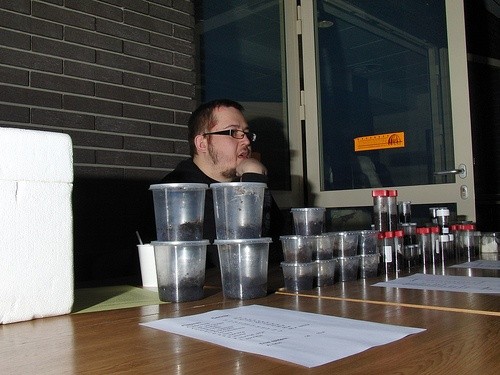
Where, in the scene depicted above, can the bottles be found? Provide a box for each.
[436,208,450,234]
[372,191,417,281]
[450,225,482,263]
[417,226,455,272]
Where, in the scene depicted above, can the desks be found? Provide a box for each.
[265,250,500,318]
[0,285,500,375]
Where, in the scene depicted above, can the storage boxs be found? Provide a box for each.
[0,126,76,326]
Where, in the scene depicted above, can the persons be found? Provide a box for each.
[130,97,296,281]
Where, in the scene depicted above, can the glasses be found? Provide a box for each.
[202,129,256,141]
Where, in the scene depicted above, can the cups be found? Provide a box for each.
[151,239,210,302]
[136,244,158,288]
[327,230,378,258]
[336,254,380,282]
[279,233,336,262]
[429,207,447,224]
[398,200,411,224]
[291,208,326,235]
[213,238,273,299]
[210,182,267,240]
[149,183,208,241]
[280,258,338,292]
